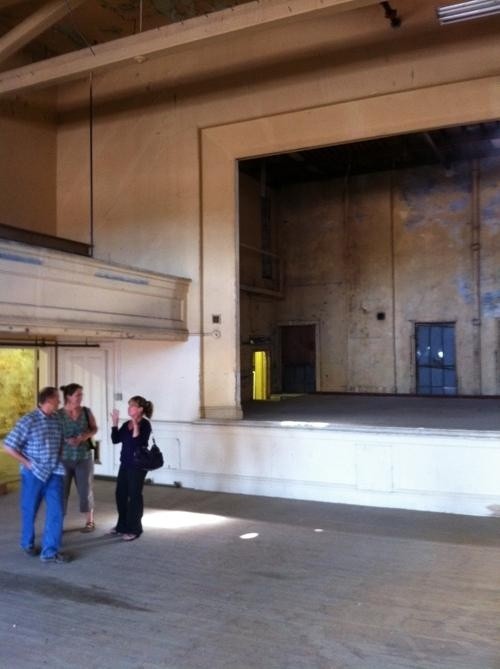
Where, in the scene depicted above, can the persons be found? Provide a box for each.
[4,387,72,562]
[59,383,99,532]
[110,396,153,540]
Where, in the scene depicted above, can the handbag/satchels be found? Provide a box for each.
[132,434,163,470]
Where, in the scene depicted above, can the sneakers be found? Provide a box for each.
[40,551,72,564]
[80,522,96,533]
[104,528,139,541]
[20,544,37,558]
